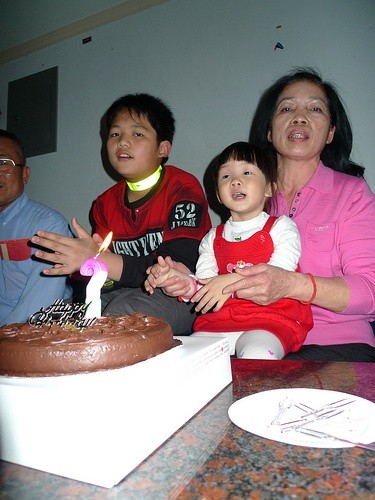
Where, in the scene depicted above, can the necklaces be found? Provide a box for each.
[124,164,163,192]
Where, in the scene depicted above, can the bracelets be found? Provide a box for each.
[300,273,317,306]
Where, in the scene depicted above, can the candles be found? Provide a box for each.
[80,257,108,318]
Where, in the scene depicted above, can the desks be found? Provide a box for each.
[0,357,375,500]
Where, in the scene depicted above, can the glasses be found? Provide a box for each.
[0,159,25,175]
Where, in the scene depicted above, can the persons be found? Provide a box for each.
[157,63,375,363]
[0,129,73,326]
[30,92,212,335]
[144,140,315,359]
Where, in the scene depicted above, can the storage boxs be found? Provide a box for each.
[0,335,233,488]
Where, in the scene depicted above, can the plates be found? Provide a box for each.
[228,388,375,448]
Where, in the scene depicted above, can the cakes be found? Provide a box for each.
[0,298,181,378]
